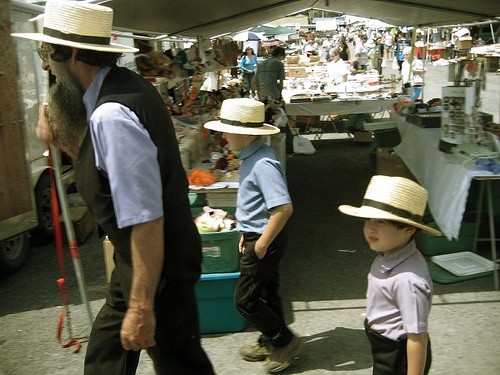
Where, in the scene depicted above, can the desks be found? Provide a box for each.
[391,101,500,291]
[284,100,396,139]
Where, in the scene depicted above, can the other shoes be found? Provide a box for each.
[240,334,277,362]
[267,337,305,374]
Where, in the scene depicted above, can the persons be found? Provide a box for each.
[337,173,444,375]
[11,1,217,375]
[299,20,424,73]
[397,46,425,86]
[203,97,306,374]
[255,46,286,123]
[324,47,348,88]
[237,44,259,98]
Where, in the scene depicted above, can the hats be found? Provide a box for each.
[9,0,140,53]
[203,99,279,135]
[338,175,443,238]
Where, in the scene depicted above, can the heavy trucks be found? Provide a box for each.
[0,0,184,273]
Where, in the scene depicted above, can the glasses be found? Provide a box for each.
[38,48,70,61]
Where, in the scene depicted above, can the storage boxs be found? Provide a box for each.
[193,231,242,274]
[59,205,93,246]
[196,274,249,334]
[376,146,410,179]
